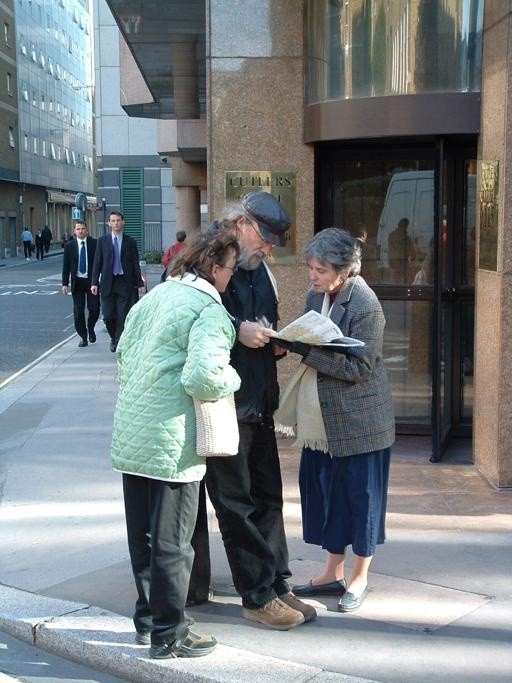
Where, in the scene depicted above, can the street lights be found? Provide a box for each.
[100,196,107,235]
[71,82,96,194]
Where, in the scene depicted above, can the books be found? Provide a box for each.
[266,310,364,348]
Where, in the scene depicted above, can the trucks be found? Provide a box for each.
[374,170,476,288]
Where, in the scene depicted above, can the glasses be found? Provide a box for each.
[247,218,277,248]
[220,264,238,273]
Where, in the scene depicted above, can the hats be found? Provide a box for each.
[240,191,292,246]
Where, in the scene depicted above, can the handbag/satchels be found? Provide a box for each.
[161,267,167,281]
[193,394,240,457]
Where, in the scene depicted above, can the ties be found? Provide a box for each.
[112,236,120,276]
[79,241,86,274]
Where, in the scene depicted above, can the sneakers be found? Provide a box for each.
[278,590,317,620]
[241,596,305,629]
[148,629,217,658]
[135,617,195,644]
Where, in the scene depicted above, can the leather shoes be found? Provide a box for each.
[338,585,369,611]
[110,339,117,351]
[89,330,95,342]
[79,337,87,346]
[291,577,347,596]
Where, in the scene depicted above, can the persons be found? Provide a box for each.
[112,230,246,658]
[35,229,45,260]
[90,210,146,352]
[278,226,398,611]
[20,225,33,259]
[385,216,414,283]
[61,220,102,346]
[161,230,190,267]
[351,222,377,285]
[42,223,52,252]
[165,188,318,630]
